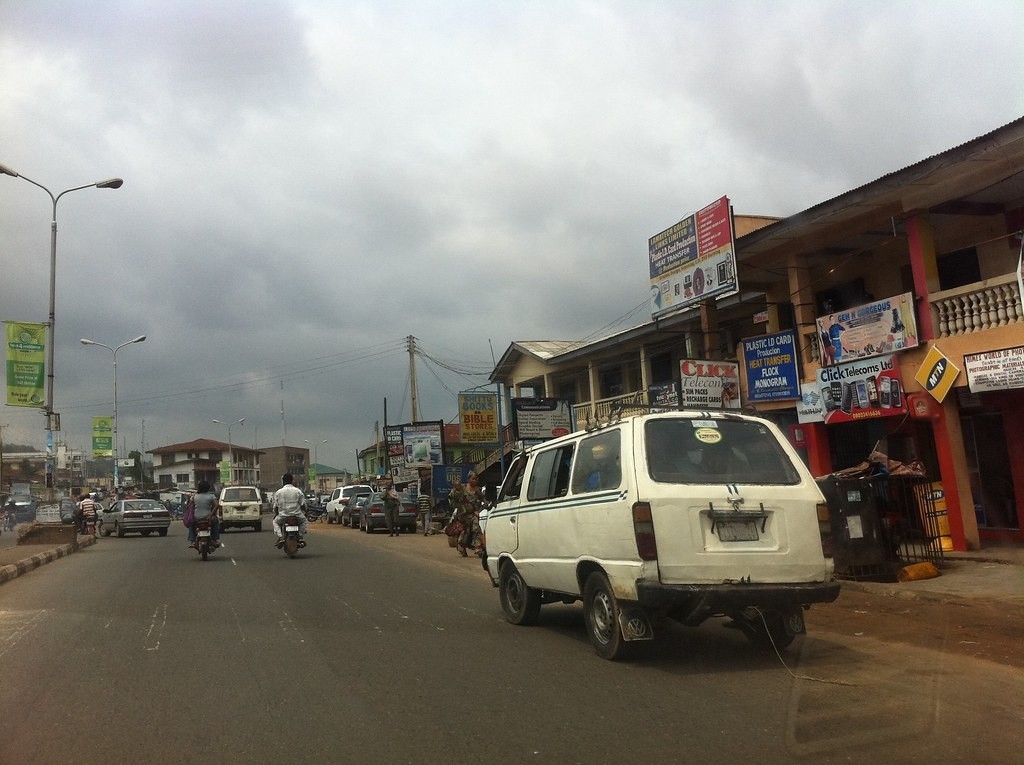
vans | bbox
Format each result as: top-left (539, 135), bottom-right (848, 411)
top-left (89, 491), bottom-right (104, 501)
top-left (479, 408), bottom-right (840, 661)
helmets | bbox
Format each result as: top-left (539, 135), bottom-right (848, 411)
top-left (84, 493), bottom-right (90, 498)
top-left (80, 493), bottom-right (84, 499)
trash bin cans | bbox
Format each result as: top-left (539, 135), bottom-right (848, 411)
top-left (817, 477), bottom-right (899, 582)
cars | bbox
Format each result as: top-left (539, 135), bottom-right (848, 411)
top-left (76, 503), bottom-right (104, 519)
top-left (309, 495), bottom-right (331, 505)
top-left (341, 494), bottom-right (370, 528)
top-left (481, 485), bottom-right (516, 503)
top-left (358, 493), bottom-right (418, 533)
top-left (98, 499), bottom-right (171, 537)
top-left (107, 489), bottom-right (117, 496)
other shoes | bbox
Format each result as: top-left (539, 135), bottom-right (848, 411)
top-left (274, 538), bottom-right (284, 547)
top-left (460, 550), bottom-right (468, 557)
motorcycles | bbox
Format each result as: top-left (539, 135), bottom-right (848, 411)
top-left (304, 503), bottom-right (328, 523)
top-left (72, 503), bottom-right (97, 539)
top-left (282, 515), bottom-right (306, 558)
top-left (192, 519), bottom-right (219, 560)
top-left (2, 511), bottom-right (16, 531)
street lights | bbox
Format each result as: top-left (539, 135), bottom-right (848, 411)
top-left (305, 439), bottom-right (328, 503)
top-left (1, 165), bottom-right (124, 521)
top-left (81, 335), bottom-right (146, 500)
top-left (212, 417), bottom-right (246, 486)
top-left (67, 448), bottom-right (79, 496)
top-left (350, 452), bottom-right (361, 485)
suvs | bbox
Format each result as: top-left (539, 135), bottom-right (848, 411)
top-left (218, 486), bottom-right (263, 532)
top-left (5, 495), bottom-right (42, 520)
top-left (323, 485), bottom-right (375, 523)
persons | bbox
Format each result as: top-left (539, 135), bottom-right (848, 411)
top-left (272, 473), bottom-right (308, 548)
top-left (675, 285), bottom-right (679, 296)
top-left (706, 274), bottom-right (712, 285)
top-left (817, 318), bottom-right (836, 366)
top-left (187, 480), bottom-right (221, 548)
top-left (93, 494), bottom-right (100, 503)
top-left (72, 493), bottom-right (98, 533)
top-left (418, 487), bottom-right (432, 536)
top-left (448, 470), bottom-right (497, 557)
top-left (898, 293), bottom-right (916, 341)
top-left (388, 443), bottom-right (402, 456)
top-left (4, 498), bottom-right (18, 525)
top-left (828, 315), bottom-right (847, 360)
top-left (379, 486), bottom-right (400, 536)
top-left (720, 371), bottom-right (739, 408)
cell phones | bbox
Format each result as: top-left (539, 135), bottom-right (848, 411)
top-left (821, 375), bottom-right (902, 415)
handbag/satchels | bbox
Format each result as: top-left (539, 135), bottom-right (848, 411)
top-left (183, 494), bottom-right (196, 528)
